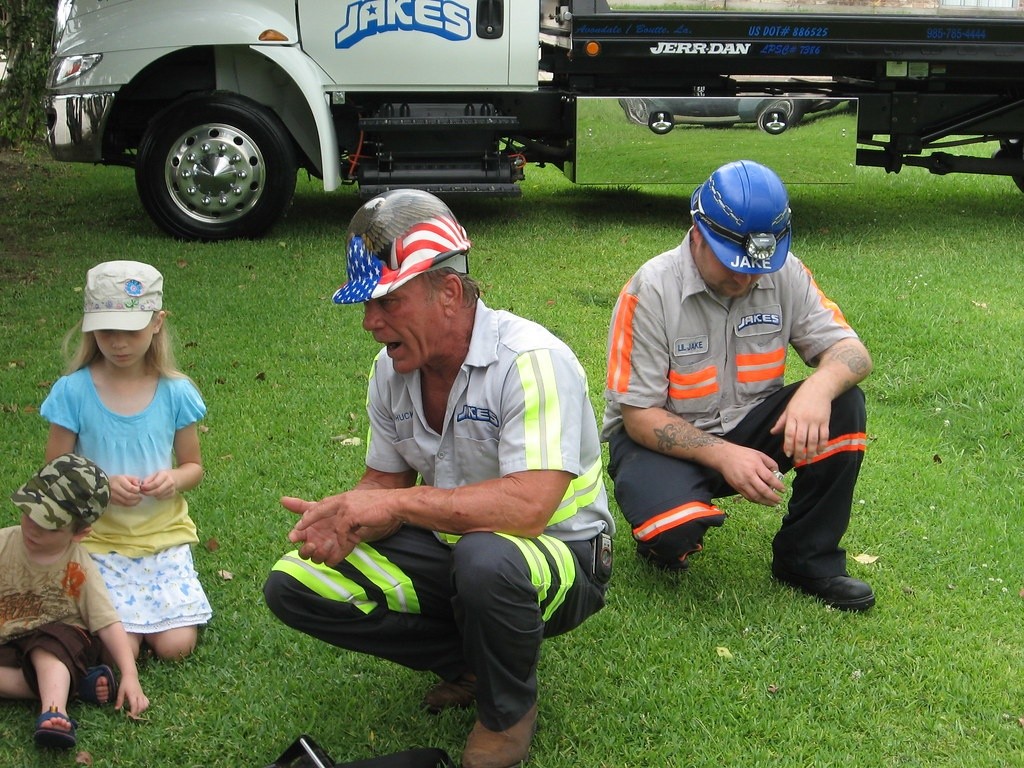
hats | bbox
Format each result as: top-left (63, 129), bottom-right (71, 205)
top-left (10, 453), bottom-right (111, 529)
top-left (80, 260), bottom-right (163, 332)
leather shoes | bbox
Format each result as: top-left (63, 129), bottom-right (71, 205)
top-left (463, 705), bottom-right (540, 768)
top-left (636, 544), bottom-right (690, 572)
top-left (771, 557), bottom-right (875, 610)
top-left (419, 668), bottom-right (478, 715)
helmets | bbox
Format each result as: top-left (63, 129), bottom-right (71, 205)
top-left (332, 187), bottom-right (472, 304)
top-left (691, 160), bottom-right (793, 273)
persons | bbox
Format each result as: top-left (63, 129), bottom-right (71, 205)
top-left (262, 188), bottom-right (616, 768)
top-left (599, 159), bottom-right (876, 612)
top-left (40, 259), bottom-right (214, 669)
top-left (1, 452), bottom-right (150, 750)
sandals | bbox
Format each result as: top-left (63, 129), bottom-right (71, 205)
top-left (34, 711), bottom-right (76, 749)
top-left (74, 665), bottom-right (116, 703)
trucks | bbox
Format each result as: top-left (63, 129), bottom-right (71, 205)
top-left (45, 0), bottom-right (1024, 242)
